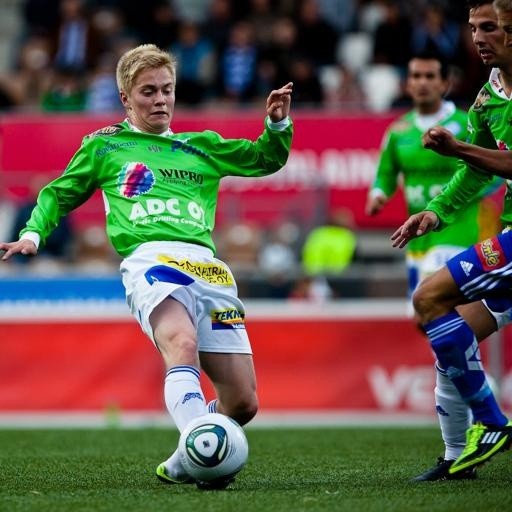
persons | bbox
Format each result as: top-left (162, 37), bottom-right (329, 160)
top-left (1, 2), bottom-right (511, 304)
top-left (408, 1), bottom-right (511, 474)
top-left (365, 45), bottom-right (503, 318)
top-left (2, 43), bottom-right (295, 490)
top-left (386, 2), bottom-right (511, 486)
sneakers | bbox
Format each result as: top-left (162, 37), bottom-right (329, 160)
top-left (448, 416), bottom-right (512, 476)
top-left (155, 447), bottom-right (192, 484)
top-left (410, 457), bottom-right (478, 481)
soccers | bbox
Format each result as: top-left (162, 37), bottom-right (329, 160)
top-left (177, 413), bottom-right (249, 482)
